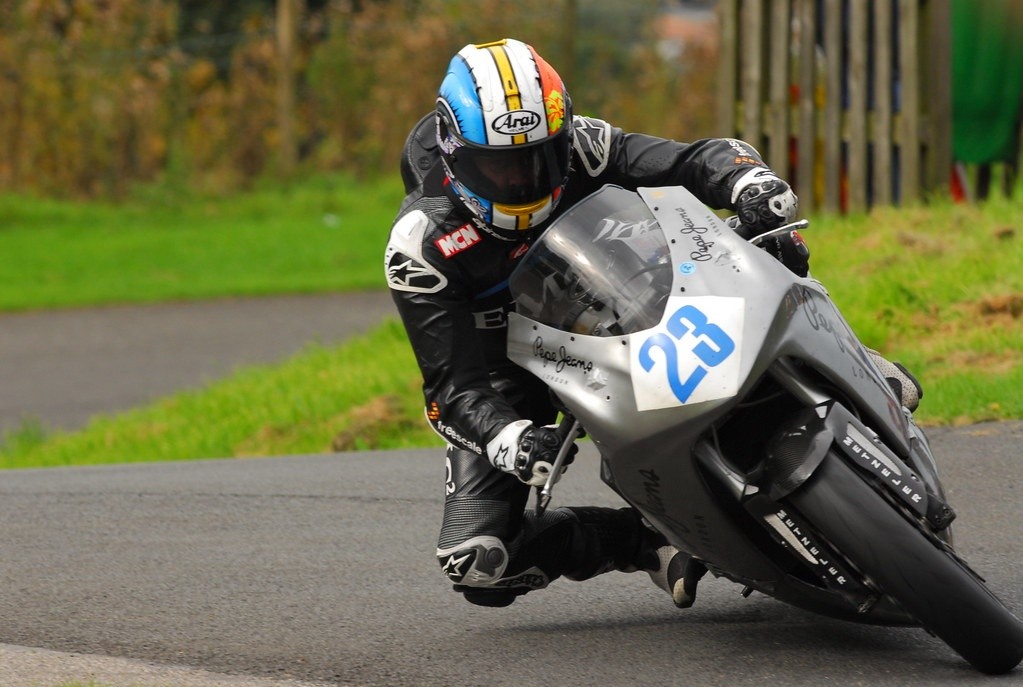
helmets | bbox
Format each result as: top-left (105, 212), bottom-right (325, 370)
top-left (435, 38), bottom-right (575, 241)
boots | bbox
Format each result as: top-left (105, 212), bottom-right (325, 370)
top-left (868, 346), bottom-right (919, 413)
top-left (554, 506), bottom-right (698, 608)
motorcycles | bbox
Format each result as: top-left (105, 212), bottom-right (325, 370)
top-left (490, 179), bottom-right (1022, 678)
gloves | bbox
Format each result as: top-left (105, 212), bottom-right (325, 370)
top-left (484, 419), bottom-right (587, 488)
top-left (727, 165), bottom-right (800, 246)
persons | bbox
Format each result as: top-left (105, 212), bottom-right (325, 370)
top-left (383, 39), bottom-right (798, 608)
